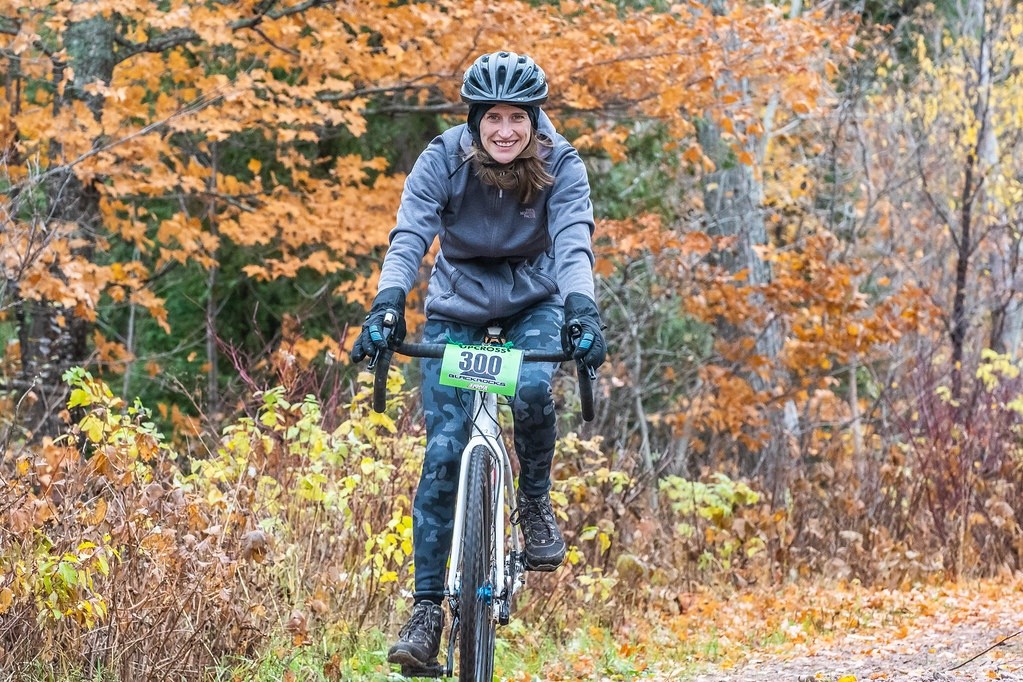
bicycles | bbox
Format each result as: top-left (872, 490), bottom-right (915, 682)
top-left (367, 308), bottom-right (608, 682)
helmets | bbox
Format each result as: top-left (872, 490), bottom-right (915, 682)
top-left (459, 51), bottom-right (549, 105)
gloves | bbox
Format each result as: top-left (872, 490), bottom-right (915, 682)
top-left (351, 287), bottom-right (407, 364)
top-left (564, 293), bottom-right (607, 371)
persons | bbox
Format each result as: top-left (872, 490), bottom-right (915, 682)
top-left (350, 50), bottom-right (610, 666)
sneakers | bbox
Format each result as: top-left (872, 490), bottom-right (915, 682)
top-left (388, 600), bottom-right (445, 667)
top-left (509, 486), bottom-right (566, 571)
